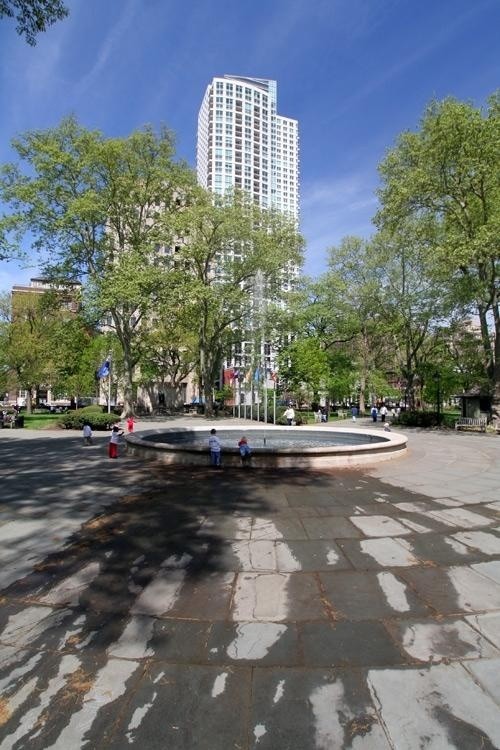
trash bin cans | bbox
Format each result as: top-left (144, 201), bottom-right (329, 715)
top-left (197, 404), bottom-right (204, 414)
top-left (14, 416), bottom-right (25, 428)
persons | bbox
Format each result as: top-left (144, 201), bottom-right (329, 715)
top-left (207, 428), bottom-right (226, 467)
top-left (31, 400), bottom-right (69, 414)
top-left (371, 410), bottom-right (378, 422)
top-left (379, 404), bottom-right (388, 422)
top-left (370, 406), bottom-right (378, 421)
top-left (0, 405), bottom-right (20, 429)
top-left (396, 407), bottom-right (401, 418)
top-left (213, 399), bottom-right (219, 417)
top-left (237, 435), bottom-right (253, 460)
top-left (351, 405), bottom-right (358, 423)
top-left (108, 426), bottom-right (125, 459)
top-left (383, 420), bottom-right (392, 433)
top-left (82, 420), bottom-right (94, 446)
top-left (283, 404), bottom-right (296, 426)
top-left (126, 415), bottom-right (136, 433)
top-left (391, 407), bottom-right (396, 418)
top-left (311, 400), bottom-right (329, 423)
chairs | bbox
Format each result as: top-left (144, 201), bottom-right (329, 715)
top-left (314, 411), bottom-right (327, 423)
top-left (337, 410), bottom-right (347, 421)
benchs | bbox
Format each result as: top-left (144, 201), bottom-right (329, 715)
top-left (455, 416), bottom-right (487, 433)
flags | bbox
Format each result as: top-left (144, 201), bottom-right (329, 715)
top-left (223, 365), bottom-right (280, 384)
top-left (96, 356), bottom-right (110, 380)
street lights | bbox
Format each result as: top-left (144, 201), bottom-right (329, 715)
top-left (432, 371), bottom-right (441, 423)
